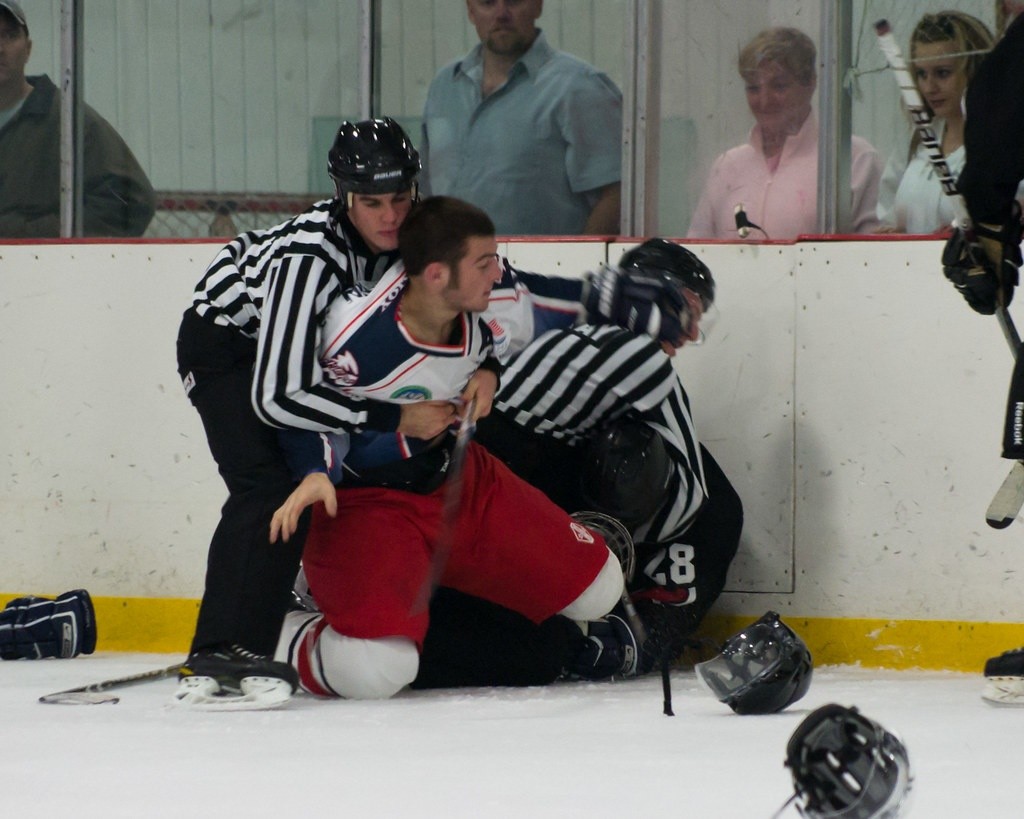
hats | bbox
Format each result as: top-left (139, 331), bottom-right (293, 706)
top-left (1, 0), bottom-right (26, 27)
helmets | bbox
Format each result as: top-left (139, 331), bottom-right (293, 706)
top-left (702, 610), bottom-right (813, 716)
top-left (619, 237), bottom-right (715, 313)
top-left (575, 416), bottom-right (670, 527)
top-left (326, 116), bottom-right (421, 196)
top-left (784, 705), bottom-right (909, 819)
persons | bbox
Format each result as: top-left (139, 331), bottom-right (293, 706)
top-left (420, 0), bottom-right (622, 235)
top-left (940, 1), bottom-right (1024, 316)
top-left (0, 0), bottom-right (158, 239)
top-left (882, 9), bottom-right (995, 234)
top-left (683, 26), bottom-right (884, 239)
top-left (268, 194), bottom-right (702, 699)
top-left (176, 115), bottom-right (502, 711)
top-left (413, 237), bottom-right (744, 689)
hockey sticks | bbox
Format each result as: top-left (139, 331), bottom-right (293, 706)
top-left (37, 662), bottom-right (185, 705)
top-left (873, 18), bottom-right (1023, 530)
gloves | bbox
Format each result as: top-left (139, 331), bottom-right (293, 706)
top-left (584, 264), bottom-right (691, 347)
top-left (0, 588), bottom-right (97, 660)
top-left (941, 200), bottom-right (1024, 316)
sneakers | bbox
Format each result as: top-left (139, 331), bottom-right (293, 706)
top-left (980, 648), bottom-right (1024, 707)
top-left (175, 638), bottom-right (300, 712)
